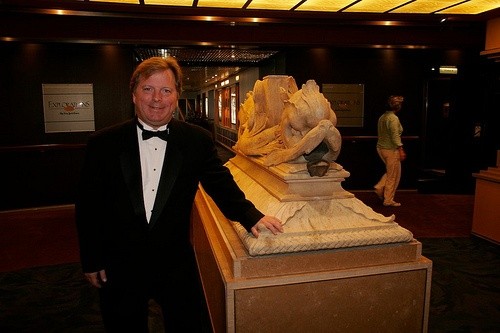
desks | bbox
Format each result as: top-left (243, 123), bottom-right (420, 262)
top-left (472, 173), bottom-right (500, 245)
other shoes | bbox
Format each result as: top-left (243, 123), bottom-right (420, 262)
top-left (384, 201), bottom-right (401, 206)
top-left (375, 185), bottom-right (382, 199)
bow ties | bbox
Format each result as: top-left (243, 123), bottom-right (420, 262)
top-left (143, 129), bottom-right (169, 141)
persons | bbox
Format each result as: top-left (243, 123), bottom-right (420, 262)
top-left (374, 94), bottom-right (405, 205)
top-left (73, 56), bottom-right (284, 332)
